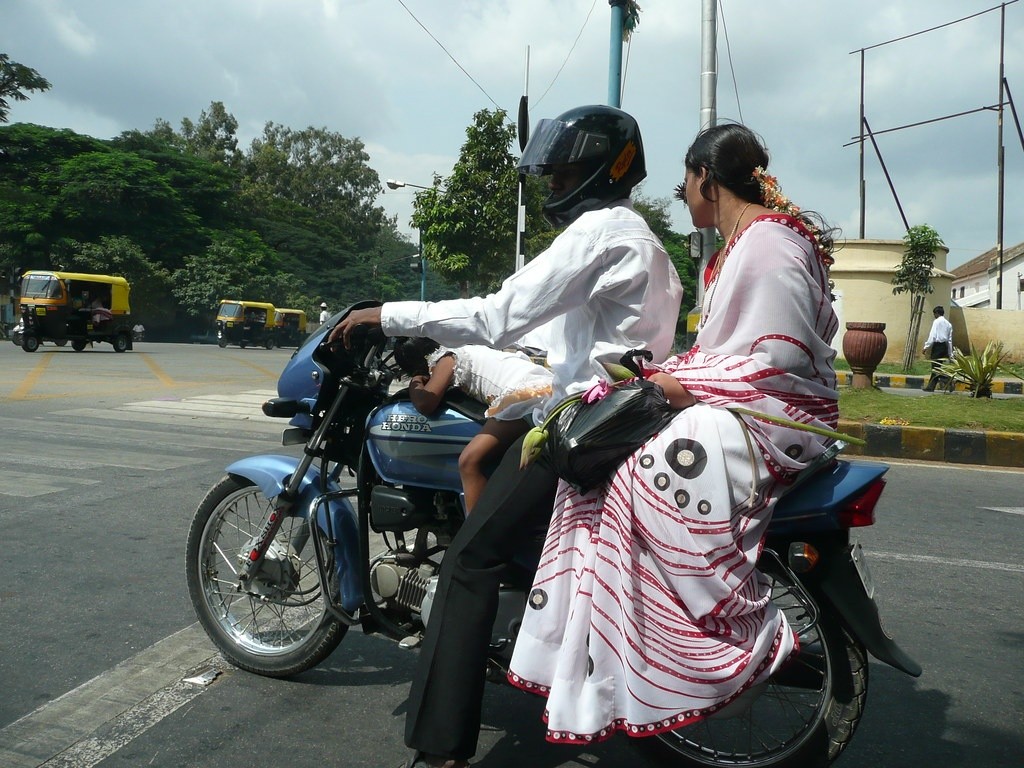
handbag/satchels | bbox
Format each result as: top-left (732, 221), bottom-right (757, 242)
top-left (536, 349), bottom-right (683, 497)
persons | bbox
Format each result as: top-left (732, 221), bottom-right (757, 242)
top-left (921, 306), bottom-right (953, 391)
top-left (325, 103), bottom-right (839, 768)
top-left (132, 320), bottom-right (145, 341)
top-left (319, 302), bottom-right (331, 326)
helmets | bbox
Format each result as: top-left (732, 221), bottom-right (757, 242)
top-left (536, 104), bottom-right (647, 229)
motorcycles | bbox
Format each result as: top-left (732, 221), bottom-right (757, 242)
top-left (274, 309), bottom-right (308, 348)
top-left (184, 300), bottom-right (924, 768)
top-left (14, 269), bottom-right (133, 353)
top-left (214, 297), bottom-right (276, 350)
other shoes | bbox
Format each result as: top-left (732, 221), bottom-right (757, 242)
top-left (922, 387), bottom-right (934, 392)
top-left (397, 749), bottom-right (468, 768)
